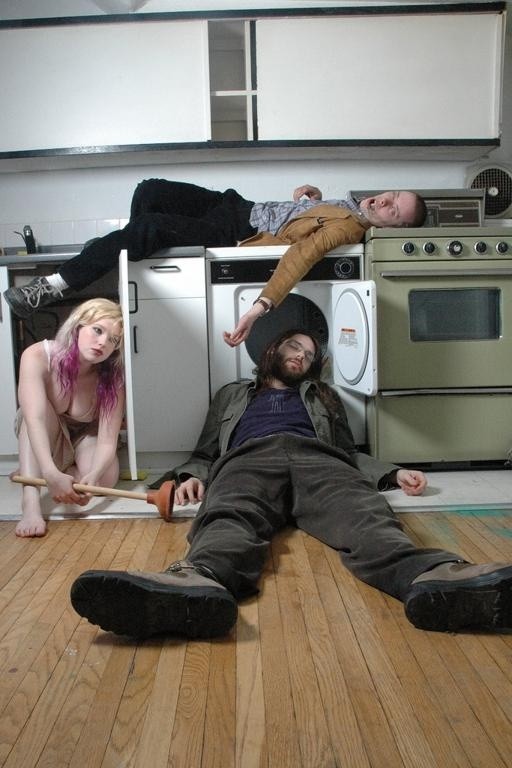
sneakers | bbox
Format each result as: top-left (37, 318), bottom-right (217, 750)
top-left (70, 558), bottom-right (238, 640)
top-left (3, 275), bottom-right (64, 319)
top-left (403, 557), bottom-right (512, 636)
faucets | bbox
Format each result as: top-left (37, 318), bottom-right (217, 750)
top-left (24, 225), bottom-right (36, 254)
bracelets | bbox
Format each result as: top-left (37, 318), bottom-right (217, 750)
top-left (253, 298), bottom-right (270, 317)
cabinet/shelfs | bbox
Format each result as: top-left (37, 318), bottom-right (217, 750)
top-left (129, 257), bottom-right (212, 470)
top-left (0, 247), bottom-right (139, 483)
top-left (0, 3), bottom-right (508, 176)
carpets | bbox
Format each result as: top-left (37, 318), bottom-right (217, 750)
top-left (0, 468), bottom-right (512, 522)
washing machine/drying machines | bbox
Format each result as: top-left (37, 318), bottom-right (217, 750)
top-left (206, 255), bottom-right (379, 446)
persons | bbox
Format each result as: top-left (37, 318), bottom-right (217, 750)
top-left (71, 328), bottom-right (512, 641)
top-left (13, 297), bottom-right (126, 538)
top-left (2, 179), bottom-right (428, 346)
